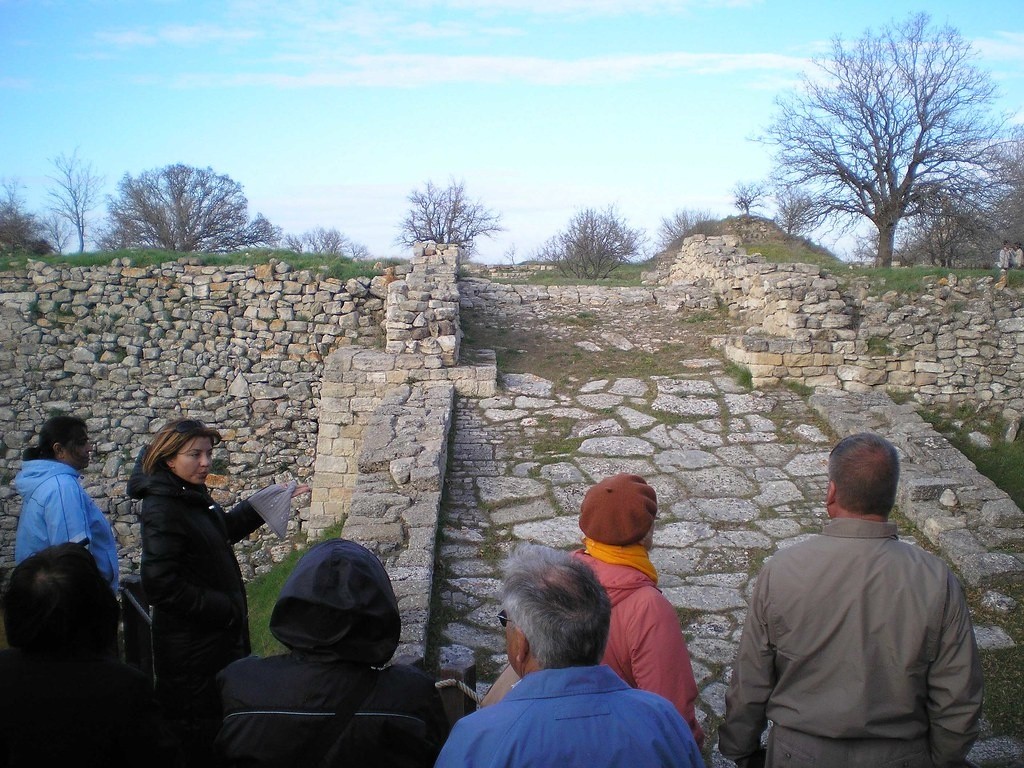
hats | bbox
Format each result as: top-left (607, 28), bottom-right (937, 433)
top-left (251, 482), bottom-right (296, 543)
top-left (579, 473), bottom-right (657, 547)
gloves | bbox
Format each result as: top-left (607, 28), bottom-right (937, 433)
top-left (736, 750), bottom-right (767, 768)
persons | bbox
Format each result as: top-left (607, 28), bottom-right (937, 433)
top-left (1, 540), bottom-right (215, 767)
top-left (996, 239), bottom-right (1024, 270)
top-left (215, 537), bottom-right (451, 767)
top-left (716, 436), bottom-right (983, 768)
top-left (568, 473), bottom-right (706, 748)
top-left (431, 543), bottom-right (706, 768)
top-left (128, 418), bottom-right (310, 740)
top-left (15, 415), bottom-right (119, 594)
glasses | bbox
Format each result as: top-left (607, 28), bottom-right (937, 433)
top-left (496, 608), bottom-right (512, 626)
